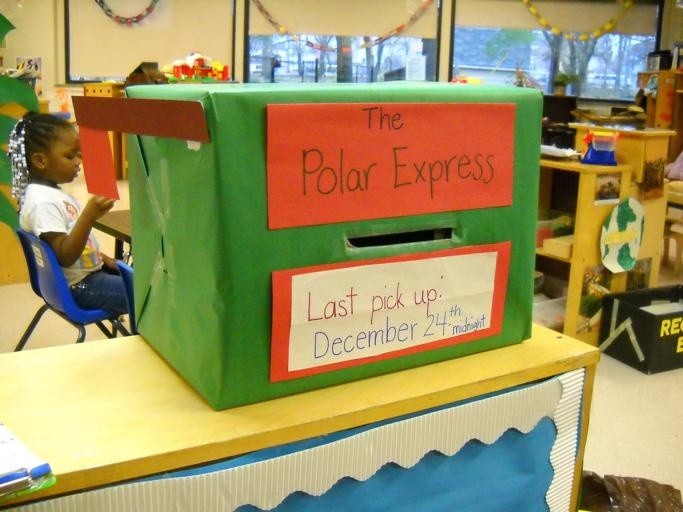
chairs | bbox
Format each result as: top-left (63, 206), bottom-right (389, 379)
top-left (15, 232), bottom-right (132, 352)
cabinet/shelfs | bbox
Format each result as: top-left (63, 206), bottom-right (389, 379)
top-left (533, 129), bottom-right (671, 355)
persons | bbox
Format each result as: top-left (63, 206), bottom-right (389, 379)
top-left (11, 112), bottom-right (134, 316)
